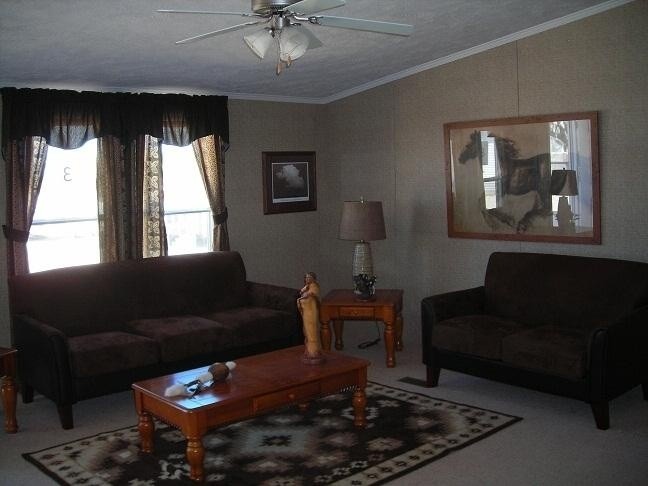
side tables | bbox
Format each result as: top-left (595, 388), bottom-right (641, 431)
top-left (319, 288), bottom-right (404, 368)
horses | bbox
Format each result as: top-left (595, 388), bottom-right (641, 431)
top-left (458, 130), bottom-right (567, 234)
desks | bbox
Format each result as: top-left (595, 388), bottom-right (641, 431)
top-left (1, 346), bottom-right (18, 435)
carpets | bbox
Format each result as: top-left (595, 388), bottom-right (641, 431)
top-left (21, 378), bottom-right (524, 486)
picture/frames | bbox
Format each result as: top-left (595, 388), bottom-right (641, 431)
top-left (261, 150), bottom-right (317, 216)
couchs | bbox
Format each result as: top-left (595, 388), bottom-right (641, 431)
top-left (419, 254), bottom-right (647, 430)
top-left (9, 253), bottom-right (302, 430)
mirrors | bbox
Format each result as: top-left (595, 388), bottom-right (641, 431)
top-left (443, 112), bottom-right (601, 244)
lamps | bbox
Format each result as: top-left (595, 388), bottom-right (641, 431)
top-left (548, 167), bottom-right (579, 232)
top-left (336, 195), bottom-right (387, 302)
top-left (244, 0), bottom-right (310, 74)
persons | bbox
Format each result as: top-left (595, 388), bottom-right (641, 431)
top-left (296, 271), bottom-right (326, 365)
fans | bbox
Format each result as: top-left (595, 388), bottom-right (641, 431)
top-left (156, 0), bottom-right (410, 51)
